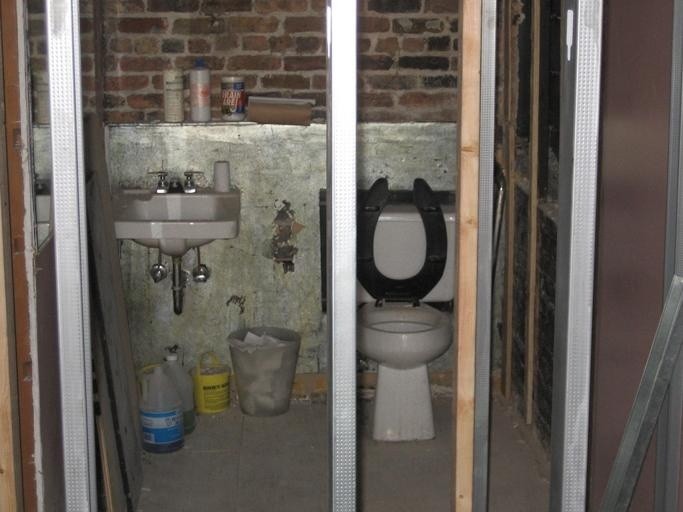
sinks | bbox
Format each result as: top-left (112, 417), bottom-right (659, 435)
top-left (33, 221), bottom-right (55, 251)
top-left (110, 185), bottom-right (242, 256)
top-left (35, 193), bottom-right (53, 224)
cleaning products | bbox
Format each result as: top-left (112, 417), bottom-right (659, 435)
top-left (161, 56), bottom-right (186, 123)
top-left (188, 58), bottom-right (213, 123)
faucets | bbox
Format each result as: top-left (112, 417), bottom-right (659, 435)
top-left (167, 178), bottom-right (184, 193)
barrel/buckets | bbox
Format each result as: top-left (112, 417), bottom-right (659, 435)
top-left (137, 364), bottom-right (187, 453)
top-left (163, 344), bottom-right (198, 435)
top-left (190, 349), bottom-right (231, 417)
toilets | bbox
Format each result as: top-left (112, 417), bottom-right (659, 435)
top-left (357, 177), bottom-right (457, 444)
